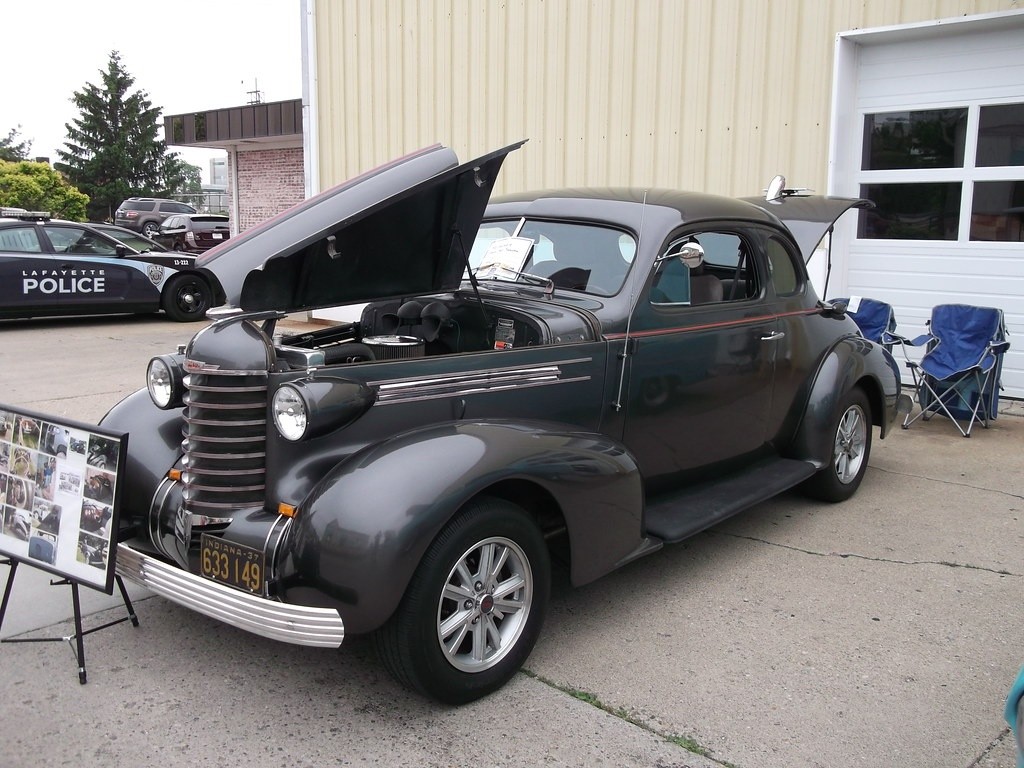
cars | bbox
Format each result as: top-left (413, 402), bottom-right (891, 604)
top-left (0, 206), bottom-right (225, 324)
top-left (84, 140), bottom-right (903, 708)
top-left (151, 213), bottom-right (230, 253)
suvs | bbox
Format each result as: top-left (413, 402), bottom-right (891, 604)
top-left (114, 197), bottom-right (198, 239)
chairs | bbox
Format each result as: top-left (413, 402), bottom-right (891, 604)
top-left (880, 304), bottom-right (1012, 438)
top-left (652, 236), bottom-right (724, 302)
top-left (941, 212), bottom-right (1024, 241)
top-left (826, 297), bottom-right (897, 354)
top-left (527, 230), bottom-right (599, 284)
